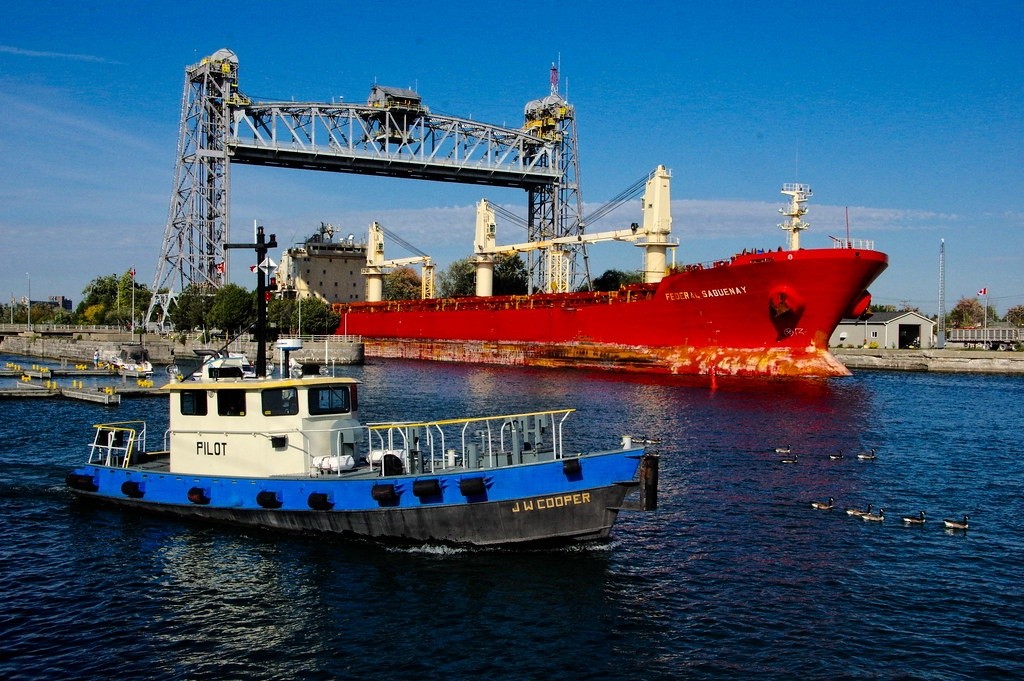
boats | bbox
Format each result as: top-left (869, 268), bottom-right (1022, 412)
top-left (61, 222), bottom-right (662, 550)
top-left (332, 164), bottom-right (892, 377)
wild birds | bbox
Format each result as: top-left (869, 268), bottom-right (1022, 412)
top-left (808, 495), bottom-right (971, 530)
top-left (770, 443), bottom-right (878, 466)
top-left (615, 434), bottom-right (664, 458)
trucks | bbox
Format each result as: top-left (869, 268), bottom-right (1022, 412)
top-left (950, 328), bottom-right (1024, 351)
